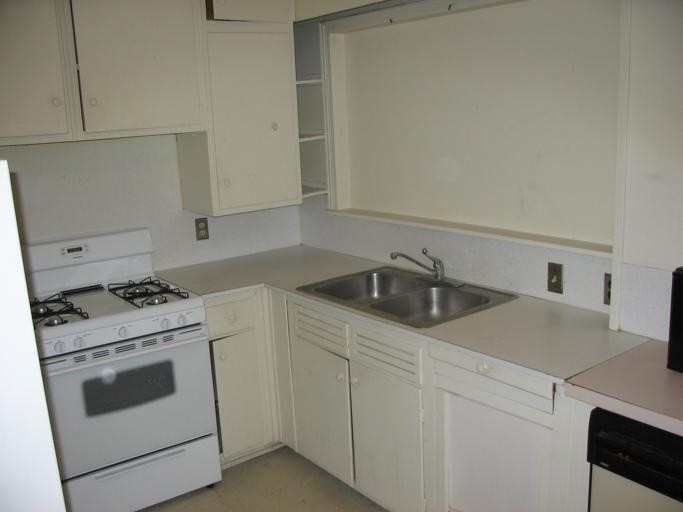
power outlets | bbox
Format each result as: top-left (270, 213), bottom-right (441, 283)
top-left (195, 216), bottom-right (208, 241)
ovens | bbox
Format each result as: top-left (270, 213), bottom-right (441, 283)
top-left (36, 323), bottom-right (225, 512)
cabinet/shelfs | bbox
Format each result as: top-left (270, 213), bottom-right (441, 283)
top-left (176, 29), bottom-right (302, 217)
top-left (205, 288), bottom-right (276, 465)
top-left (288, 295), bottom-right (425, 511)
top-left (0, 1), bottom-right (201, 142)
top-left (429, 345), bottom-right (568, 511)
top-left (295, 78), bottom-right (329, 198)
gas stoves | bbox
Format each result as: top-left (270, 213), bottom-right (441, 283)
top-left (23, 274), bottom-right (202, 331)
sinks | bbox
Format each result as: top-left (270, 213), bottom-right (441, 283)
top-left (366, 282), bottom-right (492, 327)
top-left (307, 265), bottom-right (423, 305)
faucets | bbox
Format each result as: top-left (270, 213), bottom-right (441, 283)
top-left (390, 244), bottom-right (447, 280)
